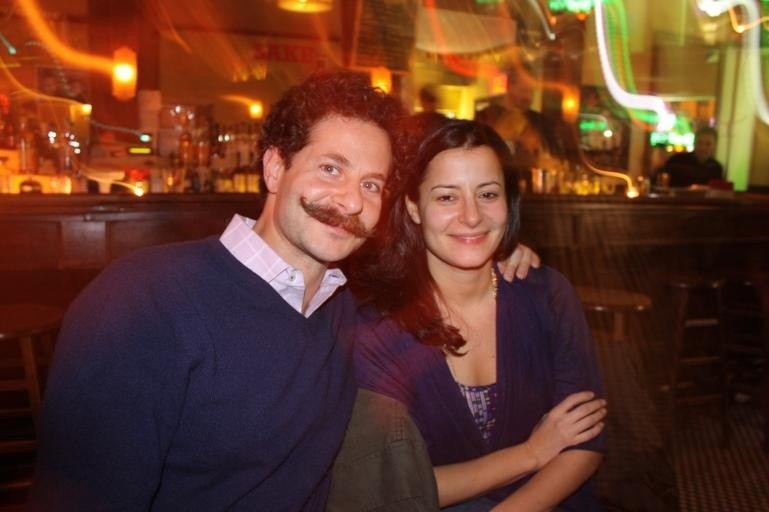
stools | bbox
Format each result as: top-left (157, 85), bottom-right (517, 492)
top-left (574, 263), bottom-right (769, 468)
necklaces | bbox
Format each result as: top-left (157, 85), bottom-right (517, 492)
top-left (489, 265), bottom-right (497, 300)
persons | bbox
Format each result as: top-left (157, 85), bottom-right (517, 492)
top-left (410, 79), bottom-right (450, 135)
top-left (651, 126), bottom-right (722, 188)
top-left (22, 70), bottom-right (541, 509)
top-left (475, 61), bottom-right (563, 152)
top-left (350, 120), bottom-right (609, 510)
